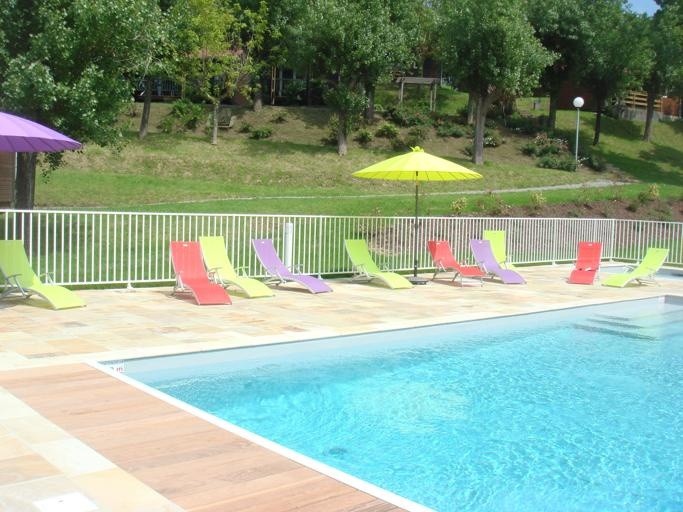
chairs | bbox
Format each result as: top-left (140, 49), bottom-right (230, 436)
top-left (470, 239), bottom-right (527, 284)
top-left (344, 239), bottom-right (415, 289)
top-left (0, 239), bottom-right (87, 310)
top-left (251, 239), bottom-right (334, 294)
top-left (567, 241), bottom-right (603, 284)
top-left (170, 241), bottom-right (233, 306)
top-left (429, 240), bottom-right (487, 288)
top-left (482, 230), bottom-right (515, 270)
top-left (602, 248), bottom-right (670, 287)
top-left (198, 235), bottom-right (275, 298)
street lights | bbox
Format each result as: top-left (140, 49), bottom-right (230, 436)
top-left (574, 97), bottom-right (585, 162)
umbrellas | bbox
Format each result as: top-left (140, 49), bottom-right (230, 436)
top-left (351, 145), bottom-right (484, 277)
top-left (0, 111), bottom-right (81, 157)
